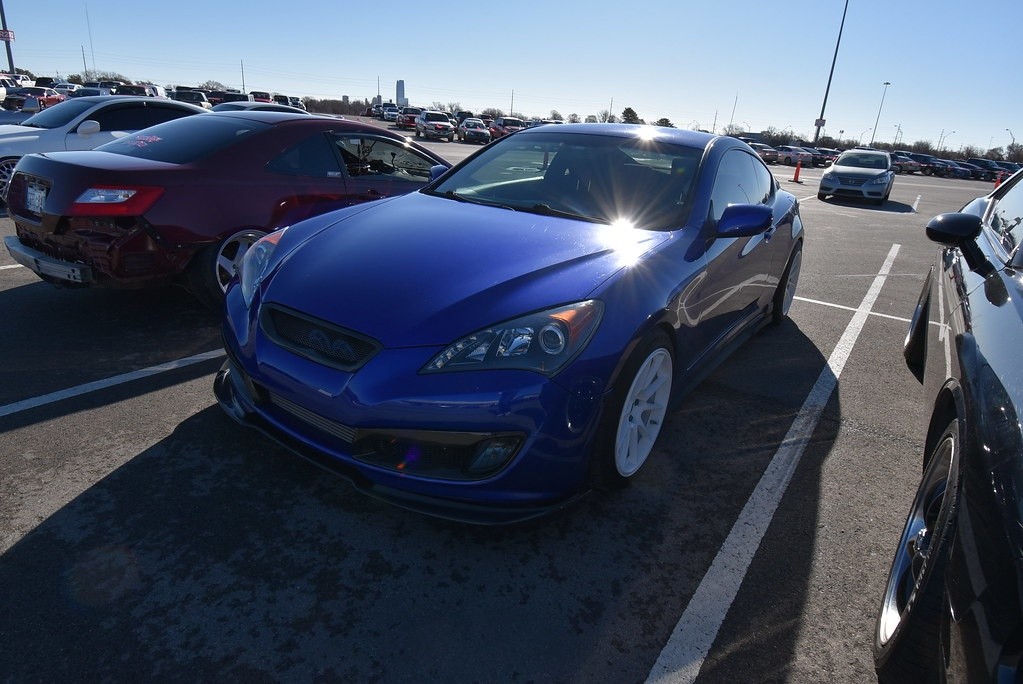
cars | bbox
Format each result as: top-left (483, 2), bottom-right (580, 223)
top-left (801, 146), bottom-right (842, 168)
top-left (872, 166), bottom-right (1023, 684)
top-left (2, 87), bottom-right (67, 110)
top-left (771, 145), bottom-right (814, 167)
top-left (890, 151), bottom-right (1023, 181)
top-left (746, 143), bottom-right (779, 165)
top-left (0, 72), bottom-right (308, 110)
top-left (0, 95), bottom-right (214, 207)
top-left (817, 148), bottom-right (900, 207)
top-left (3, 111), bottom-right (479, 320)
top-left (489, 117), bottom-right (527, 140)
top-left (371, 102), bottom-right (565, 131)
top-left (457, 119), bottom-right (490, 144)
top-left (208, 99), bottom-right (344, 119)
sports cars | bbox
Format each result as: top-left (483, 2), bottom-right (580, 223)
top-left (210, 121), bottom-right (806, 530)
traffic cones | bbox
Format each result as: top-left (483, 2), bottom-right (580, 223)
top-left (988, 175), bottom-right (1001, 196)
top-left (788, 159), bottom-right (805, 186)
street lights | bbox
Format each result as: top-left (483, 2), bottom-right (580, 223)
top-left (938, 130), bottom-right (956, 153)
top-left (1005, 129), bottom-right (1015, 160)
top-left (742, 121), bottom-right (750, 134)
top-left (780, 125), bottom-right (793, 135)
top-left (870, 82), bottom-right (891, 145)
top-left (893, 125), bottom-right (904, 146)
top-left (858, 127), bottom-right (872, 146)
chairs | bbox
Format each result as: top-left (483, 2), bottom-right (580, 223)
top-left (623, 169), bottom-right (680, 230)
top-left (874, 160), bottom-right (884, 167)
top-left (671, 158), bottom-right (697, 184)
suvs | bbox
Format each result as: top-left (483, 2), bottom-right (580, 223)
top-left (414, 111), bottom-right (455, 142)
